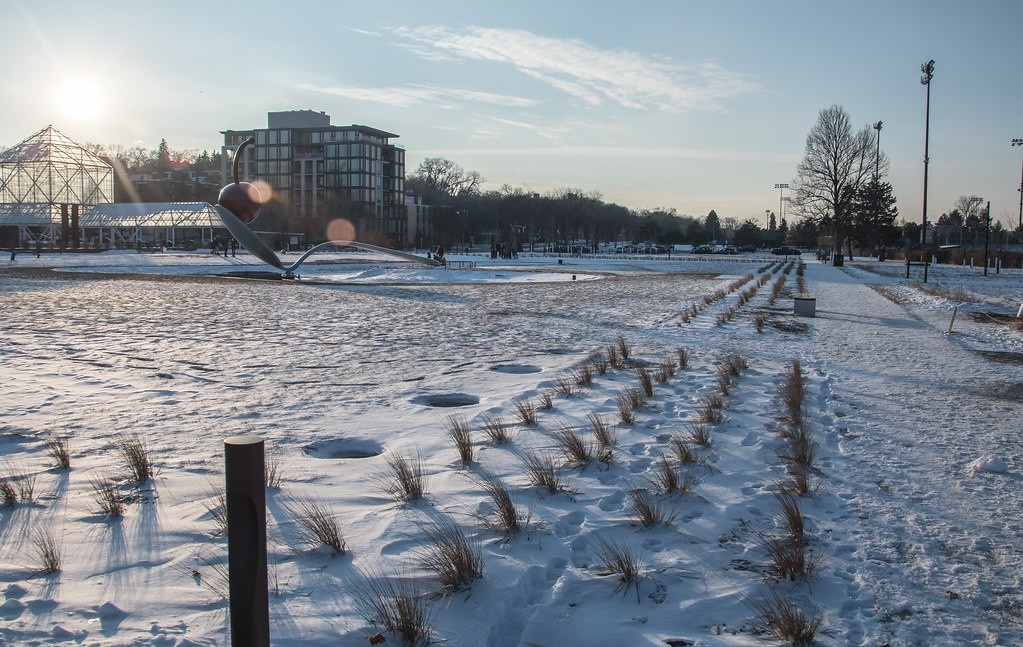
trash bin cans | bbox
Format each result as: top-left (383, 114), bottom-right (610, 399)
top-left (834, 255), bottom-right (844, 267)
top-left (491, 249), bottom-right (497, 259)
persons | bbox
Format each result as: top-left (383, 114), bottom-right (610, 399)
top-left (427, 241), bottom-right (446, 265)
top-left (383, 235), bottom-right (393, 249)
top-left (584, 240), bottom-right (599, 254)
top-left (211, 234), bottom-right (235, 257)
top-left (664, 241), bottom-right (671, 253)
top-left (491, 239), bottom-right (518, 260)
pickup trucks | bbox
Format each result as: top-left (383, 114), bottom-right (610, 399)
top-left (771, 247), bottom-right (803, 256)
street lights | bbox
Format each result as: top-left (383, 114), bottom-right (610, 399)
top-left (1011, 138), bottom-right (1023, 236)
top-left (873, 119), bottom-right (883, 236)
top-left (920, 57), bottom-right (938, 249)
top-left (775, 183), bottom-right (789, 233)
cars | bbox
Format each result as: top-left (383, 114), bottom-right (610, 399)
top-left (689, 243), bottom-right (757, 254)
top-left (551, 241), bottom-right (599, 254)
top-left (616, 243), bottom-right (674, 254)
